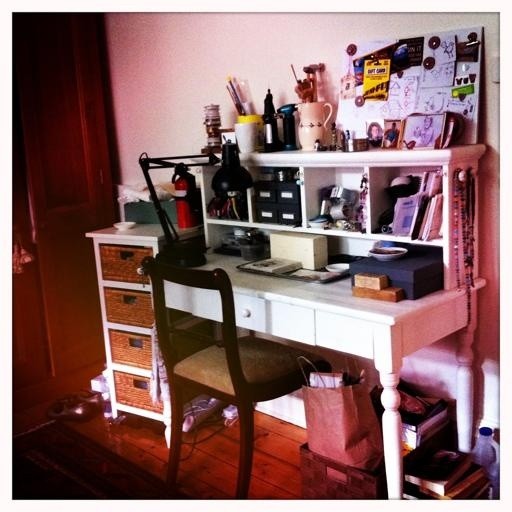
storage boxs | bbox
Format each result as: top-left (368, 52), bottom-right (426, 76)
top-left (124, 203), bottom-right (179, 224)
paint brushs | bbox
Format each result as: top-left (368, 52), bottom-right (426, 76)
top-left (225, 75), bottom-right (257, 116)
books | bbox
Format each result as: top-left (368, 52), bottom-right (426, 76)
top-left (403, 441), bottom-right (494, 498)
top-left (391, 168), bottom-right (441, 240)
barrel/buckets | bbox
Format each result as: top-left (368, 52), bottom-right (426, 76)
top-left (471, 426), bottom-right (500, 500)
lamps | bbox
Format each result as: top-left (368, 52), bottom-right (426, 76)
top-left (139, 143), bottom-right (254, 268)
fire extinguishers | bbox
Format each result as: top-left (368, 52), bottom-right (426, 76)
top-left (172, 162), bottom-right (199, 229)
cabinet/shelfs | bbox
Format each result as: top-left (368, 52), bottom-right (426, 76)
top-left (86, 222), bottom-right (180, 449)
top-left (192, 144), bottom-right (486, 500)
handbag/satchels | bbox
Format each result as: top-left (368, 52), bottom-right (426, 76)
top-left (296, 356), bottom-right (384, 484)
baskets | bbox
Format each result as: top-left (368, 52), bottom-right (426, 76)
top-left (103, 286), bottom-right (154, 328)
top-left (100, 245), bottom-right (152, 285)
top-left (112, 371), bottom-right (164, 415)
top-left (108, 329), bottom-right (153, 370)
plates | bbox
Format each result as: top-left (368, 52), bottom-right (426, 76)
top-left (325, 263), bottom-right (351, 273)
top-left (113, 221), bottom-right (136, 232)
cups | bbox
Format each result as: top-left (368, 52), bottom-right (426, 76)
top-left (351, 138), bottom-right (369, 151)
top-left (329, 203), bottom-right (352, 220)
top-left (237, 113), bottom-right (266, 153)
top-left (234, 121), bottom-right (258, 154)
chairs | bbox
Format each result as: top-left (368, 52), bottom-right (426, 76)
top-left (142, 258), bottom-right (332, 500)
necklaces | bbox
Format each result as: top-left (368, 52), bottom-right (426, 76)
top-left (452, 167), bottom-right (477, 326)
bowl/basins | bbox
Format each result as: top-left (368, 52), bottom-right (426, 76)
top-left (369, 247), bottom-right (409, 262)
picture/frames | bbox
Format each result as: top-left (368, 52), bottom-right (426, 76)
top-left (401, 112), bottom-right (446, 148)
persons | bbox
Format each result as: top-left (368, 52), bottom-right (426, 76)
top-left (416, 116), bottom-right (435, 147)
top-left (366, 120), bottom-right (383, 147)
top-left (384, 121), bottom-right (398, 148)
